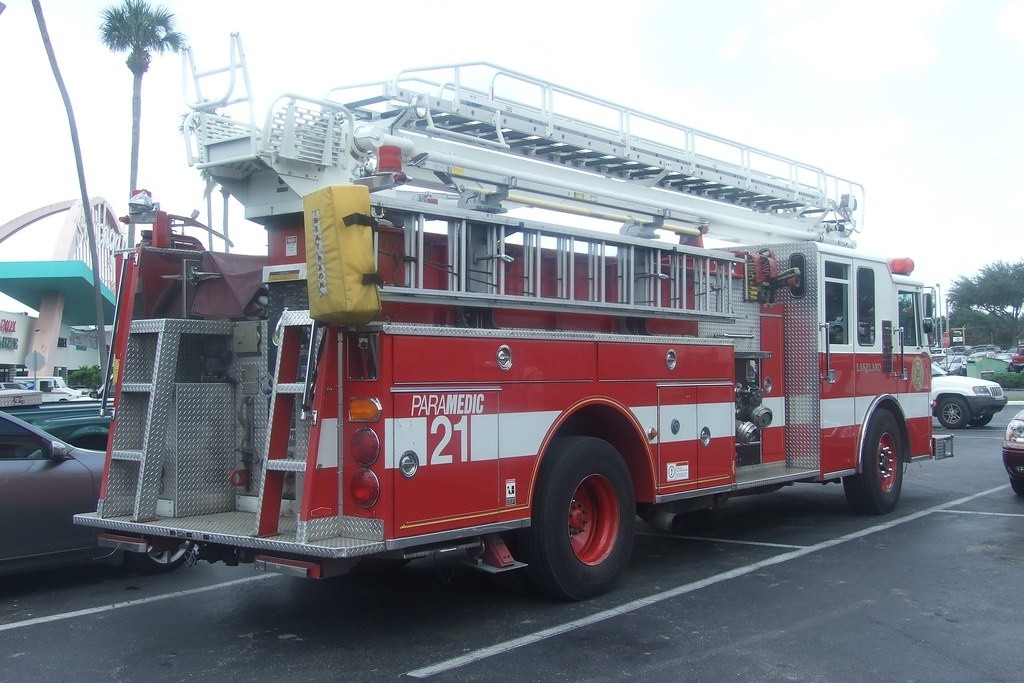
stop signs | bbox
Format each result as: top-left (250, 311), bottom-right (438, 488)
top-left (941, 332), bottom-right (950, 349)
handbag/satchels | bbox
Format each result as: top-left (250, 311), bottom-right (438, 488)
top-left (302, 184), bottom-right (382, 326)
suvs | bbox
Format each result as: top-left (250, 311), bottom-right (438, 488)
top-left (1012, 344), bottom-right (1024, 372)
top-left (930, 361), bottom-right (1008, 428)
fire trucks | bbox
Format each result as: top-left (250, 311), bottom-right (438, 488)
top-left (72, 30), bottom-right (955, 602)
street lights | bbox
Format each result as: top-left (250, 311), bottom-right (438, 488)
top-left (946, 298), bottom-right (955, 334)
top-left (936, 283), bottom-right (942, 348)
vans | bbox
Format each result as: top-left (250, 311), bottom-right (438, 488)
top-left (14, 377), bottom-right (83, 401)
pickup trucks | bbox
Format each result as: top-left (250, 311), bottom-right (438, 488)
top-left (0, 388), bottom-right (114, 451)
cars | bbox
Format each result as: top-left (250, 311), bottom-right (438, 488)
top-left (930, 344), bottom-right (1017, 376)
top-left (1002, 408), bottom-right (1024, 497)
top-left (0, 410), bottom-right (194, 573)
